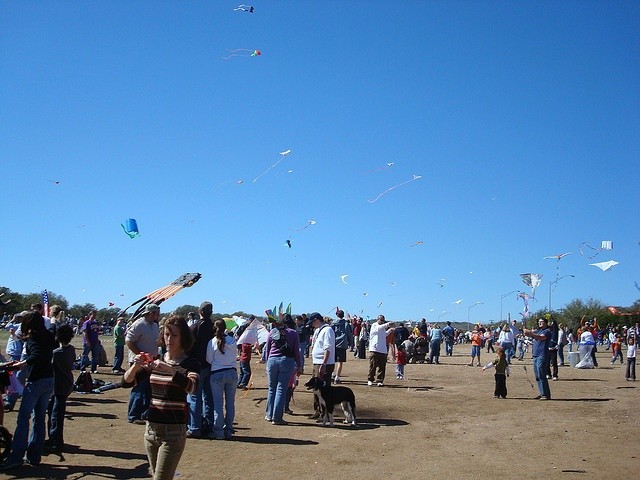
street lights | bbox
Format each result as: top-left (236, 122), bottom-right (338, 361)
top-left (270, 327), bottom-right (295, 357)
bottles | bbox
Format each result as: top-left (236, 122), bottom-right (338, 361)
top-left (306, 312), bottom-right (322, 325)
top-left (200, 301), bottom-right (213, 310)
top-left (141, 304), bottom-right (159, 316)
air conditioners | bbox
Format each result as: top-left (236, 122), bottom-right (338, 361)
top-left (304, 377), bottom-right (357, 428)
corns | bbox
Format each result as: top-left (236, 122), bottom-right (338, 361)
top-left (120, 218), bottom-right (141, 239)
top-left (279, 150), bottom-right (291, 157)
top-left (589, 260), bottom-right (619, 272)
top-left (237, 318), bottom-right (270, 353)
top-left (308, 219), bottom-right (316, 225)
top-left (174, 272), bottom-right (202, 287)
top-left (516, 291), bottom-right (535, 305)
top-left (282, 240), bottom-right (291, 248)
top-left (341, 275), bottom-right (348, 284)
top-left (601, 241), bottom-right (612, 250)
top-left (543, 252), bottom-right (572, 259)
top-left (520, 273), bottom-right (543, 292)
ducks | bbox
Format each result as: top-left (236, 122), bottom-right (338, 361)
top-left (92, 340), bottom-right (108, 367)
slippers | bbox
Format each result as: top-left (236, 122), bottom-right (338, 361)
top-left (75, 372), bottom-right (93, 392)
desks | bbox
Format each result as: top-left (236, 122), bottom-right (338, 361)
top-left (500, 291), bottom-right (520, 321)
top-left (549, 274), bottom-right (575, 311)
top-left (468, 302), bottom-right (484, 331)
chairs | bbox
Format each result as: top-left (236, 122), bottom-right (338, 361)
top-left (334, 379), bottom-right (341, 384)
top-left (208, 433), bottom-right (225, 439)
top-left (540, 395), bottom-right (551, 400)
top-left (368, 381), bottom-right (373, 386)
top-left (468, 363), bottom-right (473, 366)
top-left (23, 458), bottom-right (40, 466)
top-left (0, 455), bottom-right (23, 466)
top-left (477, 363), bottom-right (481, 366)
top-left (225, 434), bottom-right (231, 440)
top-left (283, 410), bottom-right (293, 415)
top-left (186, 431), bottom-right (204, 438)
top-left (128, 419), bottom-right (146, 425)
top-left (272, 420), bottom-right (287, 425)
top-left (265, 416), bottom-right (272, 421)
top-left (378, 382), bottom-right (384, 387)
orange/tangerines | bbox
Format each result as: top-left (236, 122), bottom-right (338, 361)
top-left (412, 336), bottom-right (431, 364)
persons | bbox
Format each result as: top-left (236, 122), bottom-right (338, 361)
top-left (491, 322), bottom-right (502, 336)
top-left (497, 323), bottom-right (515, 364)
top-left (401, 335), bottom-right (416, 364)
top-left (509, 320), bottom-right (522, 358)
top-left (121, 315), bottom-right (201, 480)
top-left (49, 304), bottom-right (64, 331)
top-left (429, 324), bottom-right (443, 364)
top-left (596, 322), bottom-right (640, 350)
top-left (577, 325), bottom-right (583, 350)
top-left (345, 313), bottom-right (362, 356)
top-left (610, 333), bottom-right (623, 364)
top-left (453, 328), bottom-right (473, 345)
top-left (416, 318), bottom-right (427, 340)
top-left (302, 313), bottom-right (312, 359)
top-left (0, 312), bottom-right (15, 327)
top-left (357, 322), bottom-right (368, 359)
top-left (523, 317), bottom-right (552, 400)
top-left (3, 311), bottom-right (31, 411)
top-left (108, 317), bottom-right (115, 335)
top-left (522, 336), bottom-right (531, 353)
top-left (57, 311), bottom-right (88, 336)
top-left (1, 310), bottom-right (56, 470)
top-left (294, 316), bottom-right (306, 375)
top-left (557, 323), bottom-right (567, 366)
top-left (265, 313), bottom-right (303, 426)
top-left (625, 328), bottom-right (637, 381)
top-left (394, 323), bottom-right (410, 345)
top-left (544, 319), bottom-right (558, 381)
top-left (515, 334), bottom-right (529, 361)
top-left (43, 325), bottom-right (76, 452)
top-left (486, 328), bottom-right (495, 353)
top-left (124, 304), bottom-right (164, 424)
top-left (580, 327), bottom-right (598, 367)
top-left (79, 310), bottom-right (100, 374)
top-left (468, 324), bottom-right (484, 367)
top-left (204, 319), bottom-right (239, 440)
top-left (185, 302), bottom-right (216, 439)
top-left (112, 317), bottom-right (128, 373)
top-left (30, 303), bottom-right (51, 330)
top-left (412, 322), bottom-right (421, 338)
top-left (568, 329), bottom-right (575, 351)
top-left (305, 312), bottom-right (335, 423)
top-left (395, 345), bottom-right (407, 379)
top-left (367, 315), bottom-right (398, 387)
top-left (442, 322), bottom-right (454, 356)
top-left (482, 346), bottom-right (510, 399)
top-left (4, 314), bottom-right (24, 376)
top-left (237, 341), bottom-right (259, 389)
top-left (100, 317), bottom-right (108, 336)
top-left (330, 310), bottom-right (354, 385)
top-left (187, 312), bottom-right (198, 327)
top-left (580, 315), bottom-right (599, 366)
